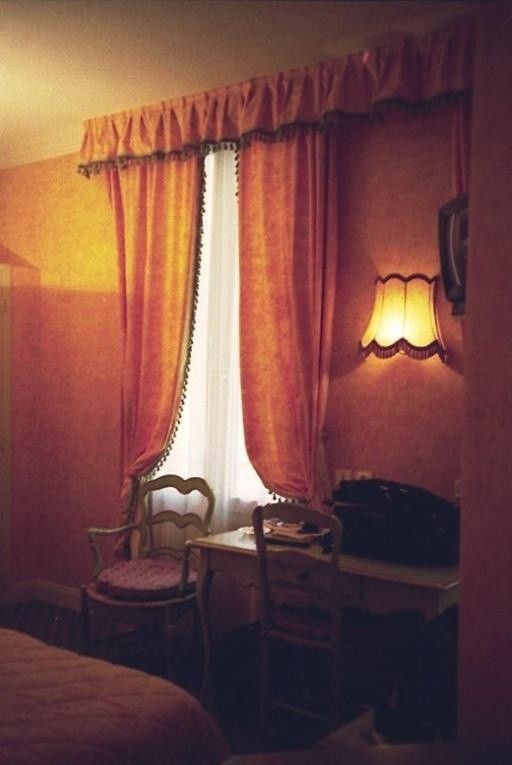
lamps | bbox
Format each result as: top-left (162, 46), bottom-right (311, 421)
top-left (356, 273), bottom-right (450, 364)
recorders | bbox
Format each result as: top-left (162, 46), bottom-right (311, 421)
top-left (326, 479), bottom-right (459, 569)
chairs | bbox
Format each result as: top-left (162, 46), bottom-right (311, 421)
top-left (77, 474), bottom-right (218, 686)
top-left (251, 502), bottom-right (397, 741)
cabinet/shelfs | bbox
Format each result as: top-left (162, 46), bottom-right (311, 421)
top-left (185, 524), bottom-right (462, 727)
